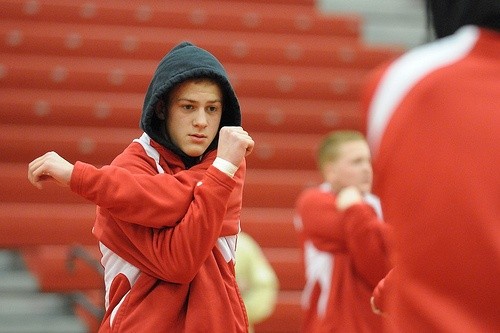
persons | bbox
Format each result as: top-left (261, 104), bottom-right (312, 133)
top-left (227, 229), bottom-right (281, 333)
top-left (26, 40), bottom-right (256, 333)
top-left (295, 126), bottom-right (394, 333)
top-left (363, 0), bottom-right (500, 333)
